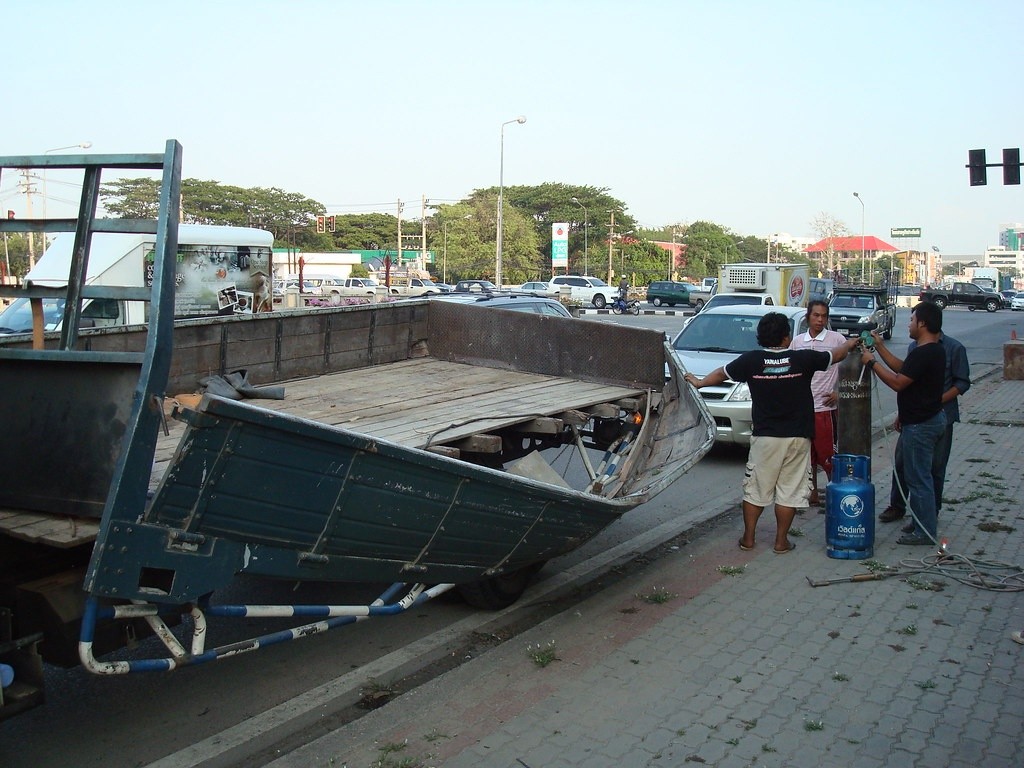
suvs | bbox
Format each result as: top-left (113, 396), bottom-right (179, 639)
top-left (646, 280), bottom-right (700, 309)
top-left (409, 276), bottom-right (621, 319)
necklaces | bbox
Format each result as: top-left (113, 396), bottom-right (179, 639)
top-left (767, 347), bottom-right (786, 349)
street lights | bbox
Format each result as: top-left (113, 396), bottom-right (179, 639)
top-left (42, 142), bottom-right (91, 254)
top-left (853, 192), bottom-right (865, 283)
top-left (571, 198), bottom-right (588, 276)
top-left (767, 233), bottom-right (778, 264)
top-left (495, 117), bottom-right (526, 290)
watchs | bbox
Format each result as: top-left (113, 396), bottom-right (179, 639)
top-left (866, 359), bottom-right (878, 370)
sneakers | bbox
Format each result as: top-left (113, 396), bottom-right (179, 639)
top-left (896, 534), bottom-right (937, 545)
top-left (901, 524), bottom-right (915, 533)
top-left (879, 506), bottom-right (906, 521)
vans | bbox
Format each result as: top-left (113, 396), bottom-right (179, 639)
top-left (809, 278), bottom-right (833, 304)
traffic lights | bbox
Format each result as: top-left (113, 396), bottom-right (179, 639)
top-left (316, 216), bottom-right (325, 233)
top-left (328, 215), bottom-right (335, 233)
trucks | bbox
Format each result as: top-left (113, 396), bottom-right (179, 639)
top-left (0, 224), bottom-right (274, 336)
top-left (825, 269), bottom-right (899, 343)
top-left (972, 268), bottom-right (1001, 293)
top-left (684, 263), bottom-right (809, 331)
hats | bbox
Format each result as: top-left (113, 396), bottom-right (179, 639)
top-left (622, 275), bottom-right (626, 278)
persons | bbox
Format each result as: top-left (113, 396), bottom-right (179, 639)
top-left (617, 275), bottom-right (629, 300)
top-left (861, 301), bottom-right (971, 544)
top-left (788, 300), bottom-right (847, 512)
top-left (685, 311), bottom-right (860, 555)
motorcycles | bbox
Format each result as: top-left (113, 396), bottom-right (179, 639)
top-left (612, 294), bottom-right (641, 316)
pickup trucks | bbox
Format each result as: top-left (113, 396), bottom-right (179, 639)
top-left (688, 282), bottom-right (717, 311)
top-left (918, 282), bottom-right (1002, 312)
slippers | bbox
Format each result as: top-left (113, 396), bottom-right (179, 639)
top-left (773, 542), bottom-right (796, 553)
top-left (808, 499), bottom-right (820, 506)
top-left (739, 537), bottom-right (752, 550)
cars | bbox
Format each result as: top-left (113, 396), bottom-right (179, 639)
top-left (274, 276), bottom-right (496, 294)
top-left (1001, 291), bottom-right (1024, 311)
top-left (664, 303), bottom-right (833, 444)
